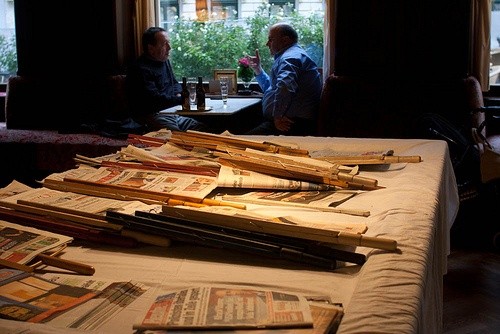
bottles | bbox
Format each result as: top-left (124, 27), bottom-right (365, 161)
top-left (196, 76), bottom-right (206, 109)
top-left (181, 77), bottom-right (190, 110)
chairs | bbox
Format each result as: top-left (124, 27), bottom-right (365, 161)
top-left (315, 75), bottom-right (347, 136)
top-left (5, 76), bottom-right (32, 130)
top-left (107, 73), bottom-right (131, 125)
top-left (466, 76), bottom-right (500, 184)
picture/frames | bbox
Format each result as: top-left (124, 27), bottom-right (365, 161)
top-left (214, 69), bottom-right (238, 95)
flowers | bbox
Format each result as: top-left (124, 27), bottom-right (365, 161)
top-left (239, 57), bottom-right (250, 68)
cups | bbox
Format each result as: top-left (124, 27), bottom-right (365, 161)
top-left (190, 93), bottom-right (196, 102)
top-left (220, 82), bottom-right (228, 104)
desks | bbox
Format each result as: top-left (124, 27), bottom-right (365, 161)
top-left (0, 135), bottom-right (459, 334)
top-left (158, 97), bottom-right (262, 117)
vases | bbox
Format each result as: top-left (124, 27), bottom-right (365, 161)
top-left (237, 67), bottom-right (256, 90)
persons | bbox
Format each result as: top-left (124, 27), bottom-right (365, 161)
top-left (125, 27), bottom-right (202, 134)
top-left (245, 23), bottom-right (322, 138)
